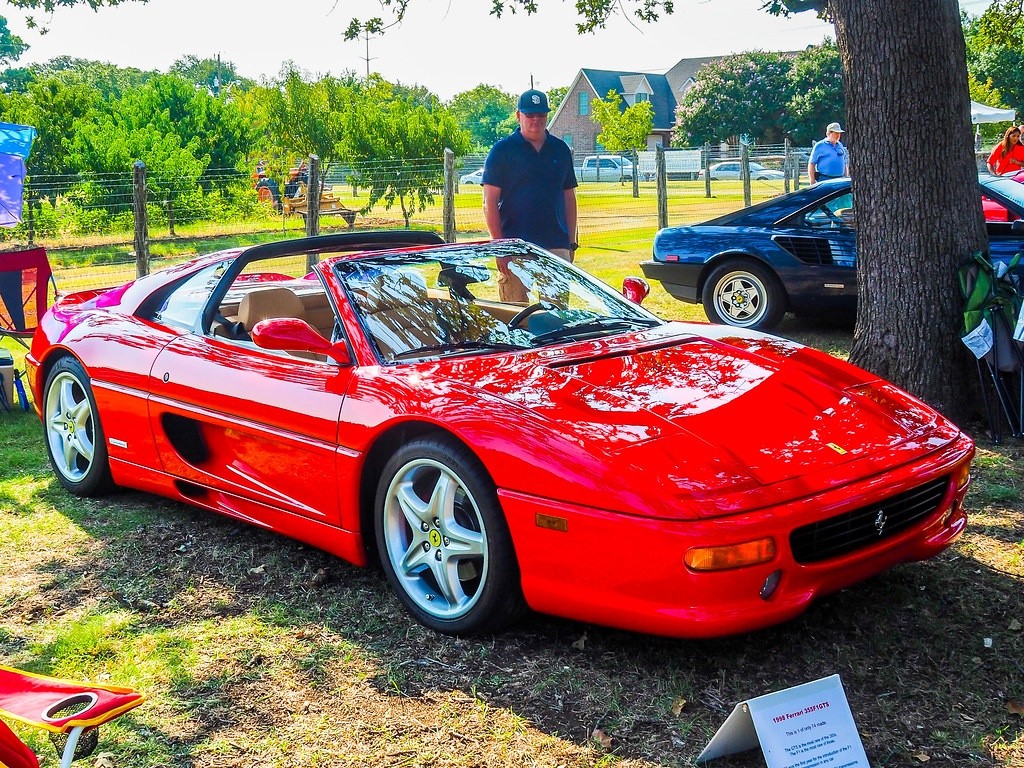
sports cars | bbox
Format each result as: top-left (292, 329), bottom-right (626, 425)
top-left (637, 172), bottom-right (1024, 333)
top-left (25, 220), bottom-right (975, 641)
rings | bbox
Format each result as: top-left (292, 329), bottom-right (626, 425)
top-left (1011, 159), bottom-right (1015, 163)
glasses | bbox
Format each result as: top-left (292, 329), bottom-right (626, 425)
top-left (525, 113), bottom-right (548, 117)
top-left (835, 148), bottom-right (845, 156)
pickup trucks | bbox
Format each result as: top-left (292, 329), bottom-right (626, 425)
top-left (571, 155), bottom-right (638, 183)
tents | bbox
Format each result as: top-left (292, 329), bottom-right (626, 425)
top-left (970, 99), bottom-right (1015, 124)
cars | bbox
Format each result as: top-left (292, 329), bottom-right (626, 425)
top-left (460, 169), bottom-right (484, 186)
top-left (698, 161), bottom-right (784, 181)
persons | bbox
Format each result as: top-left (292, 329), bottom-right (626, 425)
top-left (986, 126), bottom-right (1024, 176)
top-left (808, 122), bottom-right (849, 185)
top-left (480, 88), bottom-right (579, 310)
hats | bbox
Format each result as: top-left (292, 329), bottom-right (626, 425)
top-left (518, 89), bottom-right (552, 113)
top-left (827, 122), bottom-right (845, 133)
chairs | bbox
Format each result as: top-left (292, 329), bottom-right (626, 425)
top-left (238, 289), bottom-right (328, 363)
top-left (364, 272), bottom-right (443, 360)
top-left (831, 208), bottom-right (855, 229)
top-left (0, 247), bottom-right (56, 383)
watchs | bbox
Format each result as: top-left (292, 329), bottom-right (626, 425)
top-left (570, 243), bottom-right (578, 251)
